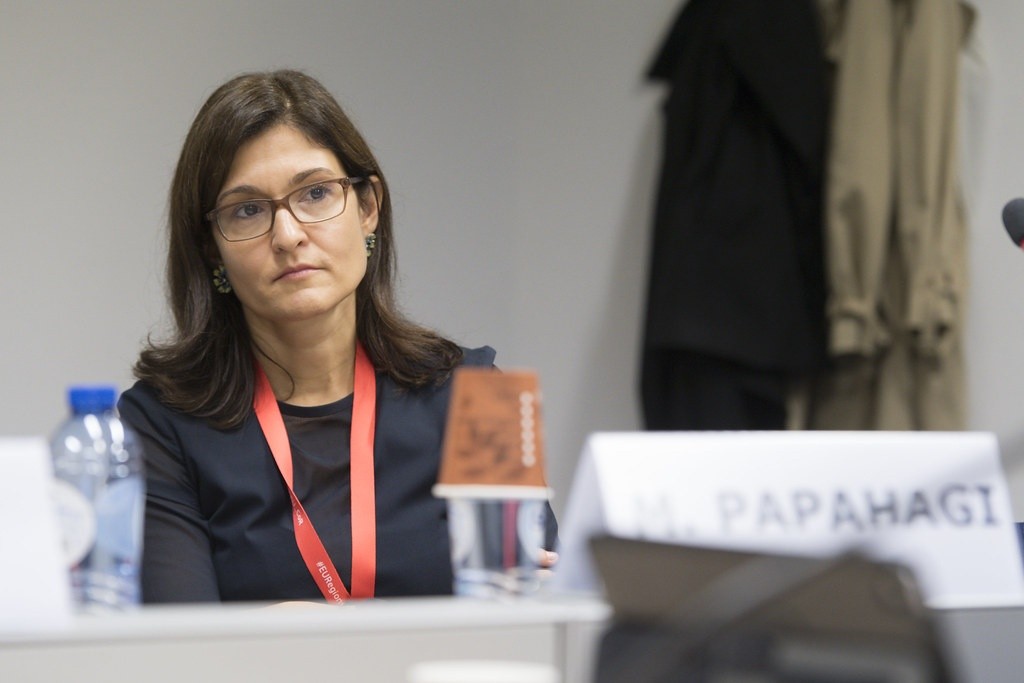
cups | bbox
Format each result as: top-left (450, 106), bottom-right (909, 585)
top-left (428, 365), bottom-right (554, 504)
top-left (442, 496), bottom-right (546, 597)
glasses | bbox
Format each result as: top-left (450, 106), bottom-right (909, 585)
top-left (206, 177), bottom-right (362, 242)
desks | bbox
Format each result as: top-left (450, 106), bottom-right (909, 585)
top-left (0, 591), bottom-right (1024, 683)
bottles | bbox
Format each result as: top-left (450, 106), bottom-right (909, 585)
top-left (46, 385), bottom-right (145, 605)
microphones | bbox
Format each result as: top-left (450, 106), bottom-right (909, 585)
top-left (1003, 198), bottom-right (1024, 252)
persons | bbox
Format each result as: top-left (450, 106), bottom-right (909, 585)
top-left (114, 67), bottom-right (560, 591)
